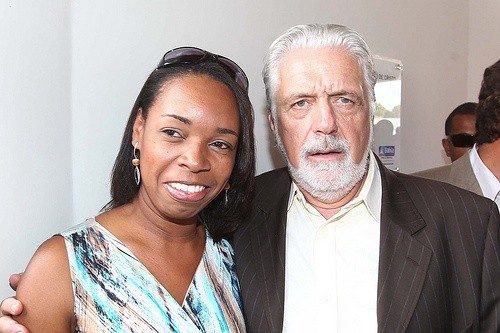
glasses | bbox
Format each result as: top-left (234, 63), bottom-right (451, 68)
top-left (447, 132), bottom-right (476, 148)
top-left (154, 46), bottom-right (249, 93)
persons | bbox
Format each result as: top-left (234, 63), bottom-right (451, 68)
top-left (406, 60), bottom-right (500, 208)
top-left (0, 23), bottom-right (500, 333)
top-left (13, 46), bottom-right (255, 333)
